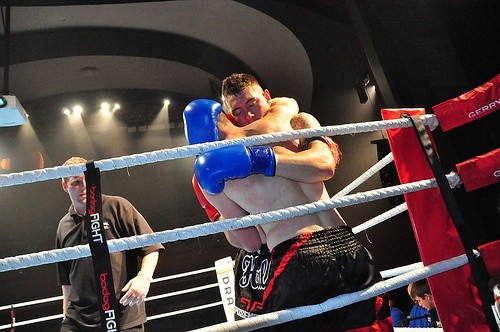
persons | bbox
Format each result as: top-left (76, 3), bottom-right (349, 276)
top-left (53, 157), bottom-right (165, 332)
top-left (181, 72), bottom-right (500, 332)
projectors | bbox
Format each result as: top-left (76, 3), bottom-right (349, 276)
top-left (0, 95), bottom-right (27, 127)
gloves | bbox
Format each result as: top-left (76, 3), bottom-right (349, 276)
top-left (194, 143), bottom-right (276, 195)
top-left (183, 99), bottom-right (222, 158)
